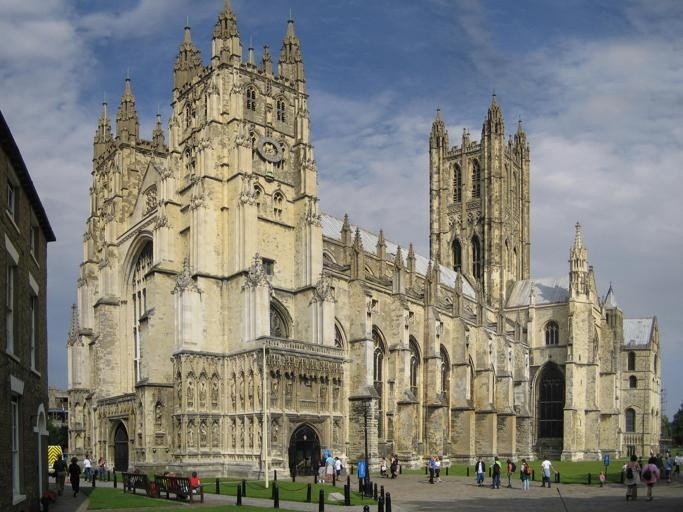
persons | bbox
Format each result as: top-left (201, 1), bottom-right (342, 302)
top-left (600, 452), bottom-right (680, 501)
top-left (98, 457), bottom-right (107, 482)
top-left (318, 455), bottom-right (326, 484)
top-left (541, 456), bottom-right (558, 488)
top-left (380, 453), bottom-right (398, 480)
top-left (127, 470), bottom-right (200, 500)
top-left (429, 455), bottom-right (442, 484)
top-left (84, 455), bottom-right (92, 484)
top-left (491, 457), bottom-right (501, 489)
top-left (507, 459), bottom-right (513, 488)
top-left (69, 458), bottom-right (81, 497)
top-left (521, 459), bottom-right (529, 491)
top-left (53, 455), bottom-right (69, 496)
top-left (326, 454), bottom-right (336, 483)
top-left (334, 457), bottom-right (342, 480)
top-left (475, 456), bottom-right (485, 487)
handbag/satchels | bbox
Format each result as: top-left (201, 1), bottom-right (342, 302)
top-left (494, 462), bottom-right (499, 474)
top-left (526, 467), bottom-right (531, 476)
top-left (643, 468), bottom-right (651, 480)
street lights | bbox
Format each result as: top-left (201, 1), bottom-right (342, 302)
top-left (360, 396), bottom-right (372, 497)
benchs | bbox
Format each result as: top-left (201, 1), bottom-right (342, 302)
top-left (152, 474), bottom-right (204, 504)
top-left (120, 472), bottom-right (153, 497)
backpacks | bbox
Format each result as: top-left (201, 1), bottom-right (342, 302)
top-left (510, 463), bottom-right (516, 472)
top-left (626, 466), bottom-right (633, 479)
